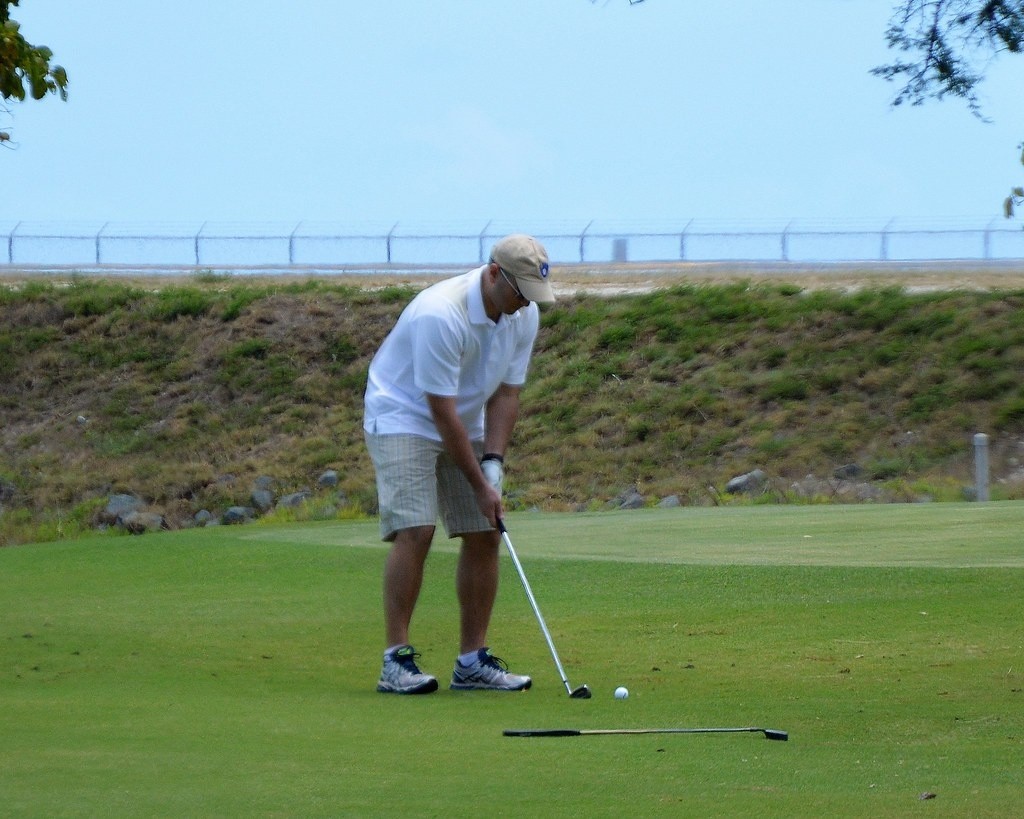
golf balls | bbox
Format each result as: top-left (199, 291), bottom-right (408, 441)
top-left (614, 686), bottom-right (629, 700)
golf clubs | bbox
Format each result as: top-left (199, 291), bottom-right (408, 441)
top-left (501, 727), bottom-right (789, 741)
top-left (495, 513), bottom-right (592, 699)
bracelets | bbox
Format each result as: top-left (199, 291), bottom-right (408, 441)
top-left (481, 452), bottom-right (503, 463)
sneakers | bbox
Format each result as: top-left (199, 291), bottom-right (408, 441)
top-left (449, 647), bottom-right (532, 691)
top-left (376, 646), bottom-right (439, 694)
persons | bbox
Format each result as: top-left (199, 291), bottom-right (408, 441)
top-left (363, 233), bottom-right (558, 695)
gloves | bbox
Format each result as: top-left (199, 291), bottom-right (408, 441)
top-left (480, 452), bottom-right (504, 499)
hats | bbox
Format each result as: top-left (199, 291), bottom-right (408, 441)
top-left (489, 234), bottom-right (556, 305)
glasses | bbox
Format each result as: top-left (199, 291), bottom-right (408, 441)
top-left (498, 268), bottom-right (526, 301)
